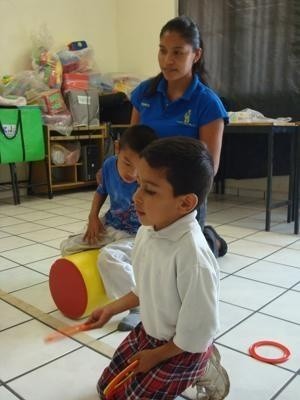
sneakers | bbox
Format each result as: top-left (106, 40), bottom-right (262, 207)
top-left (203, 224), bottom-right (229, 259)
top-left (192, 343), bottom-right (231, 400)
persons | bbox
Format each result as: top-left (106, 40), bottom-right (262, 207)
top-left (128, 14), bottom-right (228, 263)
top-left (60, 126), bottom-right (165, 331)
top-left (79, 135), bottom-right (233, 400)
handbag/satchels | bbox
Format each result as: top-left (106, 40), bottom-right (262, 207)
top-left (0, 107), bottom-right (47, 164)
top-left (64, 87), bottom-right (101, 128)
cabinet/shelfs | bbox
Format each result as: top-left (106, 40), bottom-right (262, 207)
top-left (28, 124), bottom-right (106, 198)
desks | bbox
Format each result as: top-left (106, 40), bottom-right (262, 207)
top-left (0, 105), bottom-right (46, 207)
top-left (214, 121), bottom-right (300, 232)
top-left (100, 122), bottom-right (133, 128)
